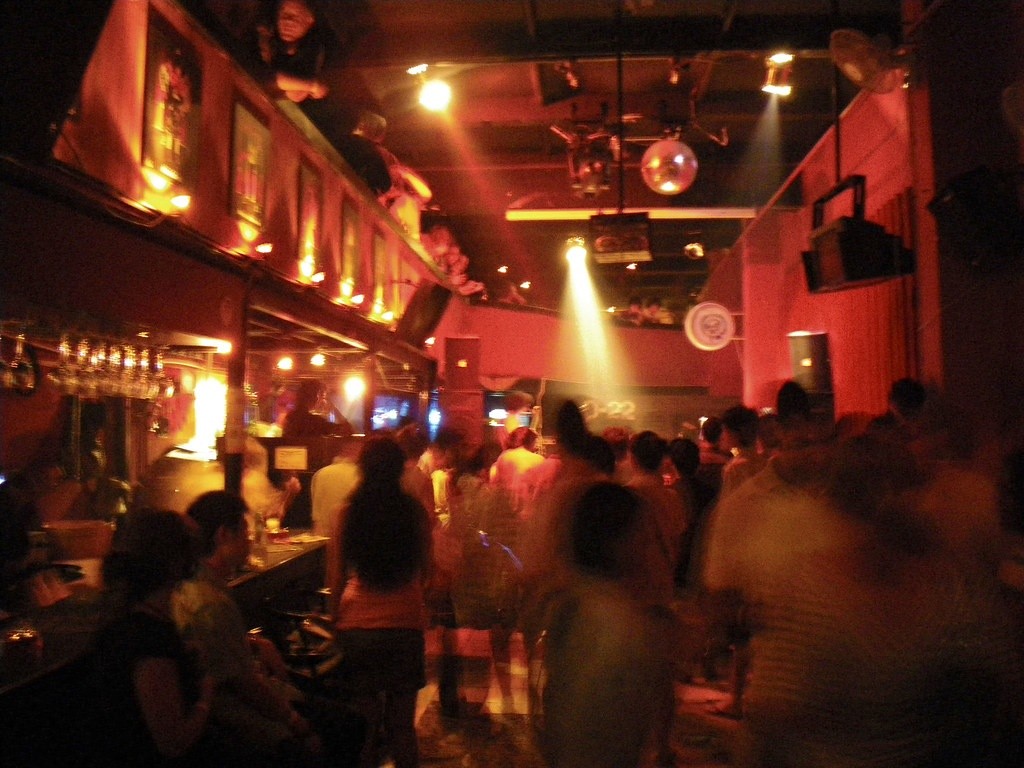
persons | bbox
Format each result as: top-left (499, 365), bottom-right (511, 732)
top-left (1, 366), bottom-right (1024, 768)
top-left (261, 0), bottom-right (329, 101)
top-left (621, 294), bottom-right (675, 325)
top-left (343, 109), bottom-right (487, 295)
